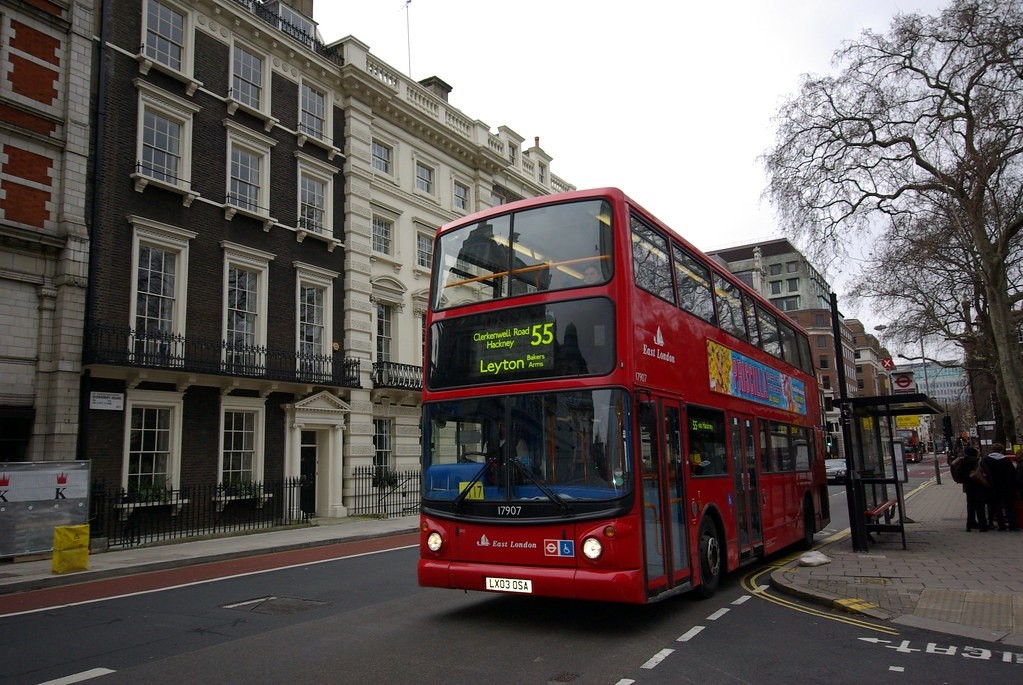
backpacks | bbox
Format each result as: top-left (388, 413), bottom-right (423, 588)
top-left (951, 458), bottom-right (968, 484)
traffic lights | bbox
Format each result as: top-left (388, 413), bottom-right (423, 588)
top-left (942, 415), bottom-right (952, 438)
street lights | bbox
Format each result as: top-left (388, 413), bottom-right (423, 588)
top-left (875, 324), bottom-right (942, 486)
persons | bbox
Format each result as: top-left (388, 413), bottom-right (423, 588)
top-left (480, 416), bottom-right (530, 486)
top-left (579, 265), bottom-right (603, 285)
top-left (953, 443), bottom-right (1023, 532)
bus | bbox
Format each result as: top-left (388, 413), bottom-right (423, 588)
top-left (895, 427), bottom-right (923, 463)
top-left (417, 186), bottom-right (831, 607)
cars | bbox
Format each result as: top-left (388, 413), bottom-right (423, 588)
top-left (824, 459), bottom-right (846, 484)
top-left (928, 440), bottom-right (949, 455)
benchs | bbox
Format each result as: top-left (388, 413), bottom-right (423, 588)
top-left (867, 498), bottom-right (897, 544)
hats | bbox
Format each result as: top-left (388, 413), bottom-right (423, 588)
top-left (965, 447), bottom-right (978, 458)
